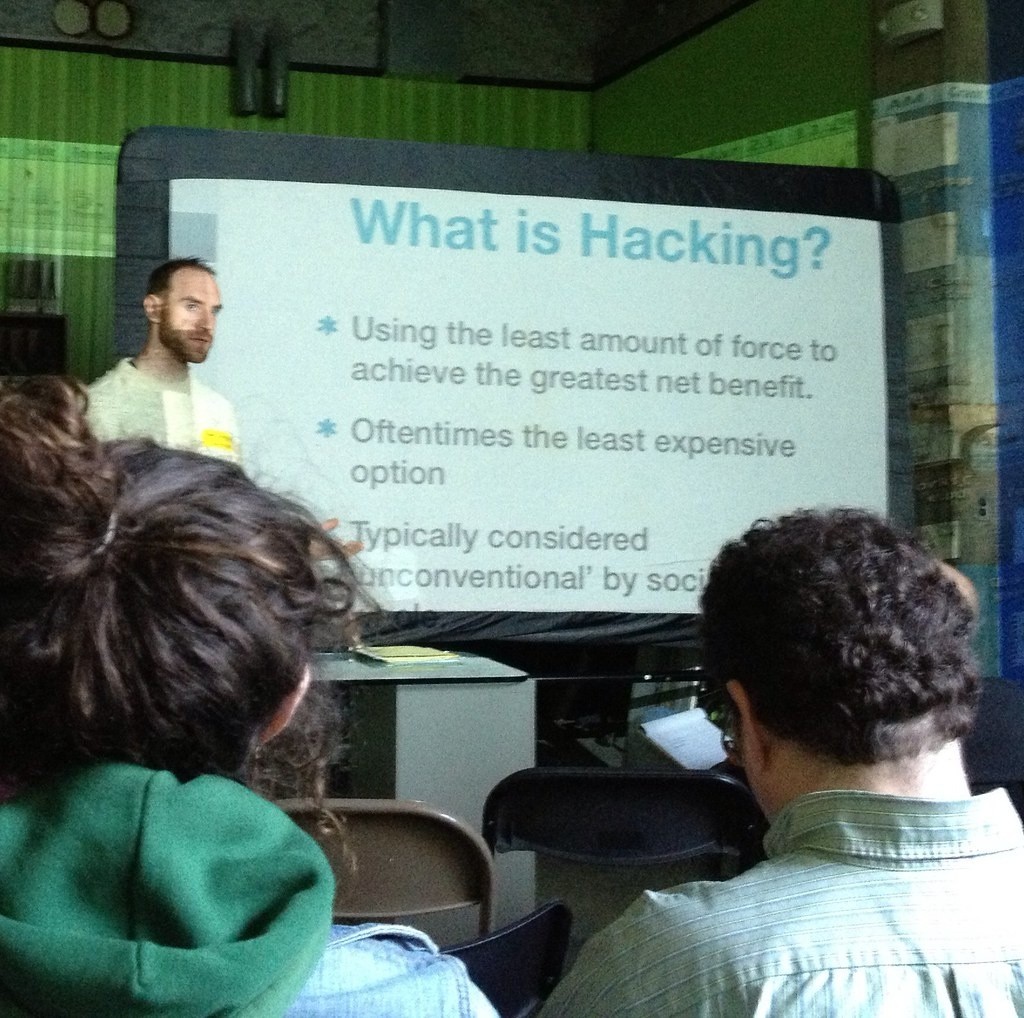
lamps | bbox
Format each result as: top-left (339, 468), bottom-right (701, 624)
top-left (50, 0), bottom-right (133, 41)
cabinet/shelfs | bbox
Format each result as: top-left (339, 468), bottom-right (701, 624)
top-left (310, 652), bottom-right (537, 947)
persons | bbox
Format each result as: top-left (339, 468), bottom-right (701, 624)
top-left (65, 255), bottom-right (242, 481)
top-left (537, 503), bottom-right (1024, 1018)
top-left (0, 380), bottom-right (513, 1018)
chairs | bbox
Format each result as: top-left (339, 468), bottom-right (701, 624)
top-left (482, 770), bottom-right (758, 877)
top-left (273, 796), bottom-right (500, 940)
top-left (440, 898), bottom-right (575, 1018)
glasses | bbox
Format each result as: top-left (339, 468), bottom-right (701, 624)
top-left (720, 698), bottom-right (746, 770)
